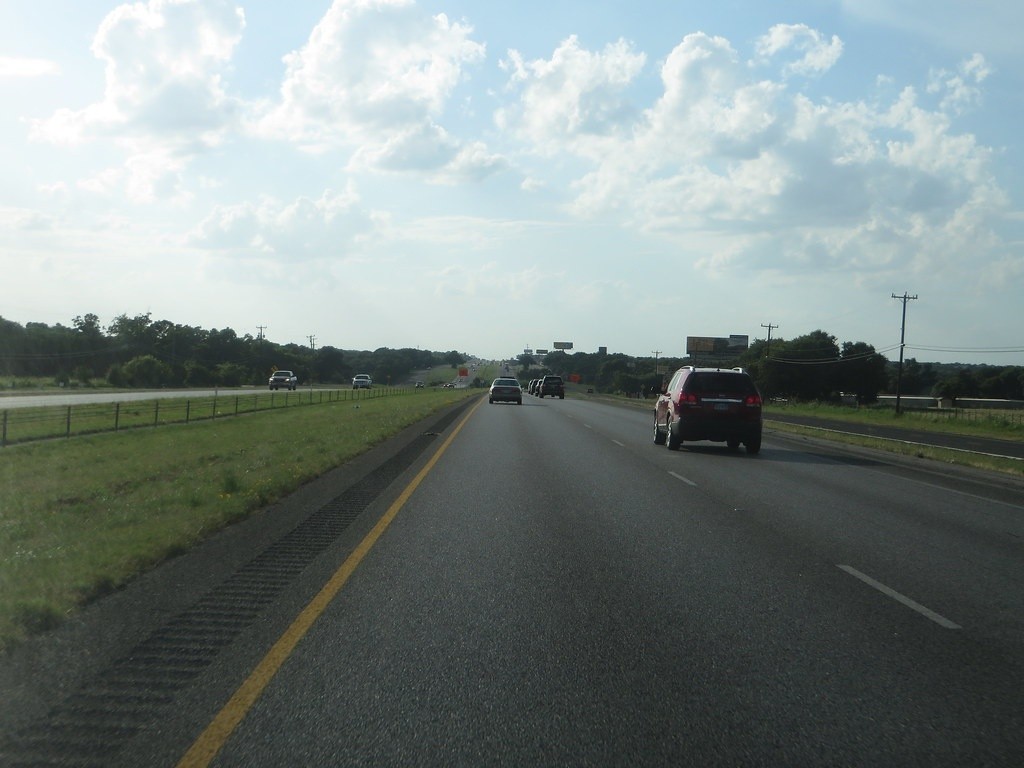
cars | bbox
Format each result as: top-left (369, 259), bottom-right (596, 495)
top-left (530, 379), bottom-right (539, 395)
top-left (489, 376), bottom-right (524, 405)
top-left (534, 379), bottom-right (543, 396)
top-left (415, 380), bottom-right (424, 388)
top-left (269, 370), bottom-right (297, 390)
top-left (528, 379), bottom-right (533, 394)
top-left (443, 381), bottom-right (457, 389)
top-left (352, 373), bottom-right (373, 390)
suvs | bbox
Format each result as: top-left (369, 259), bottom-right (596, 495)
top-left (539, 375), bottom-right (565, 399)
top-left (652, 365), bottom-right (765, 451)
